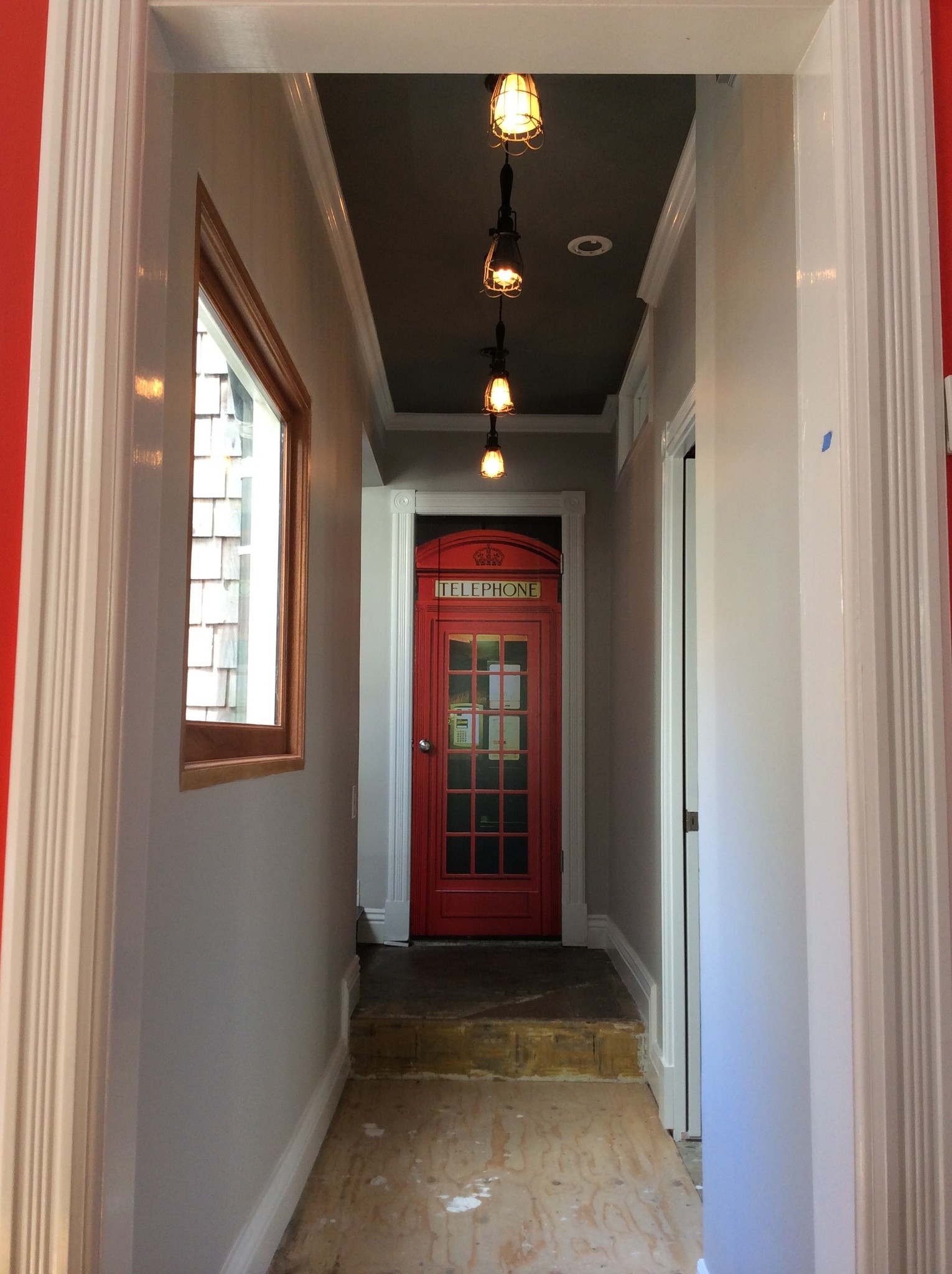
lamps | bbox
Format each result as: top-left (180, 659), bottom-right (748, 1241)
top-left (483, 73), bottom-right (545, 158)
top-left (480, 293), bottom-right (516, 418)
top-left (479, 140), bottom-right (524, 298)
top-left (478, 411), bottom-right (509, 480)
